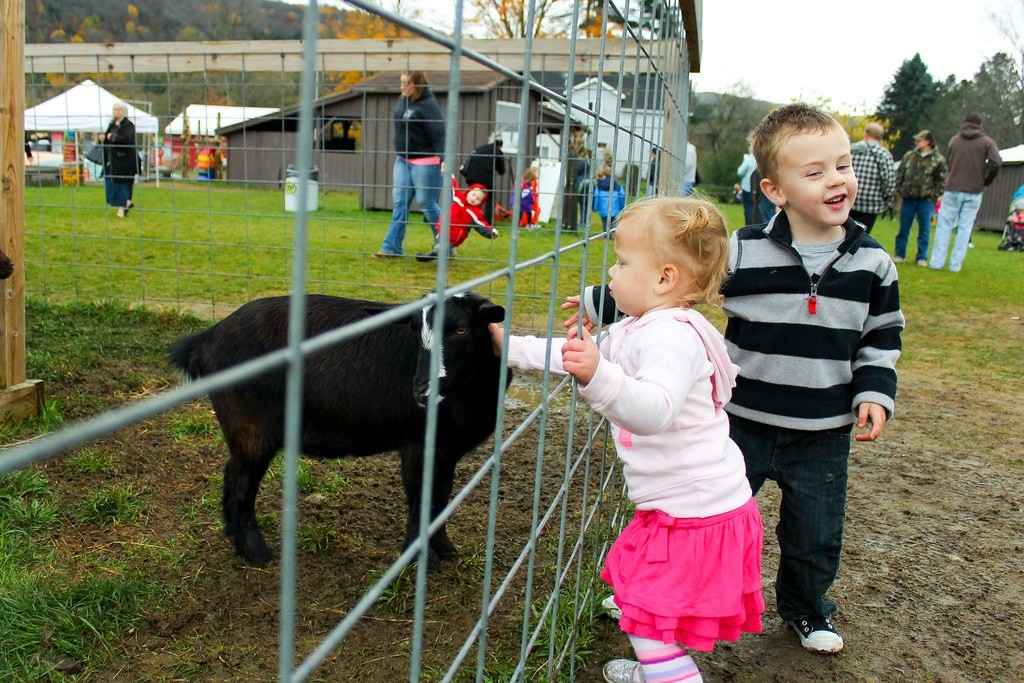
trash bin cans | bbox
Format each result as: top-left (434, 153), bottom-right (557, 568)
top-left (149, 166), bottom-right (171, 179)
top-left (284, 163), bottom-right (319, 212)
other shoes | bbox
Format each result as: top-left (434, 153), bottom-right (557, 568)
top-left (968, 242), bottom-right (974, 249)
top-left (526, 223), bottom-right (534, 230)
top-left (893, 256), bottom-right (906, 263)
top-left (603, 659), bottom-right (640, 683)
top-left (601, 595), bottom-right (622, 619)
top-left (117, 203), bottom-right (135, 218)
top-left (917, 259), bottom-right (928, 267)
top-left (370, 250), bottom-right (388, 259)
top-left (416, 252), bottom-right (438, 262)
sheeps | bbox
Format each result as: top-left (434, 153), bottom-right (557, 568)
top-left (166, 290), bottom-right (513, 578)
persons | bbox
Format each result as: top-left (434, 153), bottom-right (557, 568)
top-left (459, 140), bottom-right (505, 229)
top-left (99, 103), bottom-right (138, 220)
top-left (684, 139), bottom-right (697, 197)
top-left (562, 102), bottom-right (905, 655)
top-left (928, 112), bottom-right (1003, 273)
top-left (489, 198), bottom-right (764, 683)
top-left (733, 138), bottom-right (781, 226)
top-left (932, 190), bottom-right (1024, 248)
top-left (569, 129), bottom-right (624, 239)
top-left (370, 71), bottom-right (458, 260)
top-left (416, 174), bottom-right (499, 263)
top-left (25, 136), bottom-right (33, 161)
top-left (647, 149), bottom-right (662, 197)
top-left (882, 207), bottom-right (895, 222)
top-left (846, 123), bottom-right (896, 235)
top-left (508, 168), bottom-right (542, 230)
top-left (893, 131), bottom-right (949, 267)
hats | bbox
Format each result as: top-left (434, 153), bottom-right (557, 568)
top-left (912, 130), bottom-right (934, 142)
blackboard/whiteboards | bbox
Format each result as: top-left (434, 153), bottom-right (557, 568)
top-left (530, 159), bottom-right (566, 222)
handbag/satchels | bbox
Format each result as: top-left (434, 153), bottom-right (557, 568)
top-left (460, 150), bottom-right (476, 176)
top-left (85, 141), bottom-right (103, 166)
top-left (882, 206), bottom-right (895, 220)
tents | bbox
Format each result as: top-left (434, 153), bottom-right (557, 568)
top-left (24, 79), bottom-right (159, 191)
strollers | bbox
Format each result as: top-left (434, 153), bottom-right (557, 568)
top-left (998, 182), bottom-right (1024, 251)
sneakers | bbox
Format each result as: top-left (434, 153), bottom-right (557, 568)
top-left (788, 615), bottom-right (844, 654)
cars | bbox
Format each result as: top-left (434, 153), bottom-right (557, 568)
top-left (25, 139), bottom-right (104, 181)
